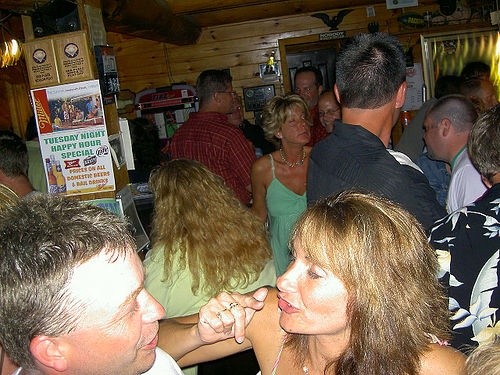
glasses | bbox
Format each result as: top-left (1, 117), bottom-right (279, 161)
top-left (212, 90), bottom-right (237, 99)
top-left (318, 107), bottom-right (341, 117)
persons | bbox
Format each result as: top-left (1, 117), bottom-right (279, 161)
top-left (0, 116), bottom-right (48, 210)
top-left (395, 61), bottom-right (498, 215)
top-left (426, 103), bottom-right (500, 355)
top-left (294, 66), bottom-right (331, 146)
top-left (319, 89), bottom-right (342, 134)
top-left (54, 95), bottom-right (103, 127)
top-left (141, 157), bottom-right (277, 375)
top-left (246, 92), bottom-right (312, 277)
top-left (455, 342), bottom-right (500, 375)
top-left (0, 194), bottom-right (185, 375)
top-left (167, 69), bottom-right (256, 208)
top-left (226, 89), bottom-right (279, 155)
top-left (156, 192), bottom-right (467, 375)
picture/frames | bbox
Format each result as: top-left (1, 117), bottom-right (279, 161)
top-left (243, 84), bottom-right (276, 112)
top-left (419, 26), bottom-right (500, 102)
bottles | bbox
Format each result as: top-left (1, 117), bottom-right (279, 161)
top-left (46, 154), bottom-right (68, 197)
top-left (266, 50), bottom-right (276, 74)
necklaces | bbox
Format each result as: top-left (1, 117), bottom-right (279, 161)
top-left (280, 147), bottom-right (306, 167)
top-left (301, 365), bottom-right (311, 375)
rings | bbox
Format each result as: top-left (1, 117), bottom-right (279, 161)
top-left (227, 302), bottom-right (239, 311)
top-left (216, 308), bottom-right (227, 317)
